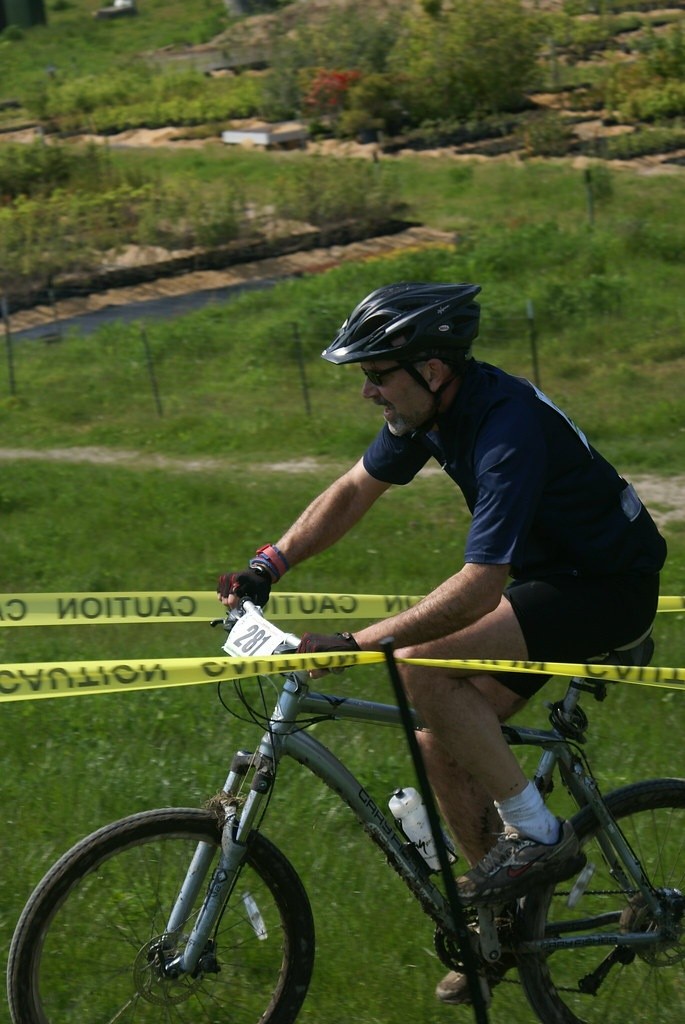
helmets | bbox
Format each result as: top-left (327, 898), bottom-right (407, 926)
top-left (321, 280), bottom-right (482, 365)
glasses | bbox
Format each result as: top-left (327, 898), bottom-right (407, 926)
top-left (360, 356), bottom-right (448, 387)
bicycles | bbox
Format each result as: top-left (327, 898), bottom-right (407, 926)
top-left (4, 586), bottom-right (685, 1024)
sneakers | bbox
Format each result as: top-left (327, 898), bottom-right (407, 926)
top-left (434, 901), bottom-right (521, 1006)
top-left (455, 816), bottom-right (587, 899)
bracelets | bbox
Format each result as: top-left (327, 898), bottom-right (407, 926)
top-left (249, 543), bottom-right (290, 584)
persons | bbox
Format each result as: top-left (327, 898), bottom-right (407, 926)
top-left (217, 283), bottom-right (669, 1005)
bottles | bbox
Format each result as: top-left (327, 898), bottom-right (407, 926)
top-left (388, 787), bottom-right (456, 871)
